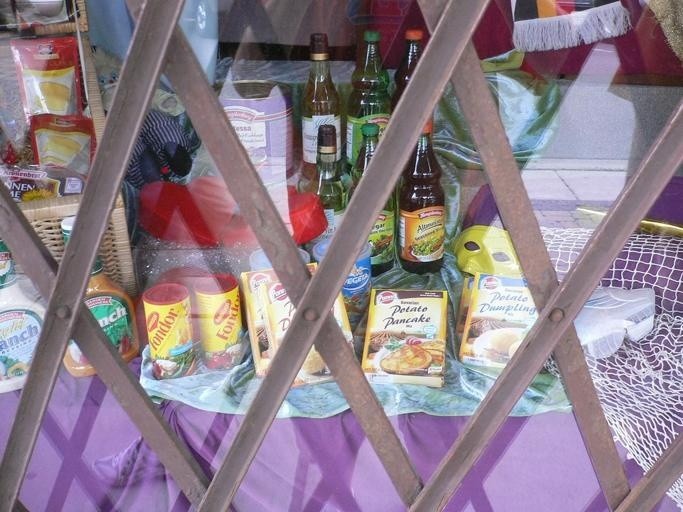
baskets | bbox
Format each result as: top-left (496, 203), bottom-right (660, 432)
top-left (0, 0), bottom-right (89, 37)
top-left (0, 1), bottom-right (140, 301)
top-left (0, 35), bottom-right (140, 301)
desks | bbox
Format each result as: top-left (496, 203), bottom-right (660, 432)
top-left (0, 57), bottom-right (683, 512)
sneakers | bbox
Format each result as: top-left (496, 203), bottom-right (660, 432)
top-left (92, 437), bottom-right (164, 486)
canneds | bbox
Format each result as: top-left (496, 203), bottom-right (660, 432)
top-left (313, 237), bottom-right (373, 335)
top-left (248, 246), bottom-right (310, 273)
top-left (141, 266), bottom-right (243, 382)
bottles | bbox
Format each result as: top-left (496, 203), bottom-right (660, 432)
top-left (51, 216), bottom-right (140, 379)
top-left (0, 237), bottom-right (49, 397)
top-left (296, 29), bottom-right (448, 289)
